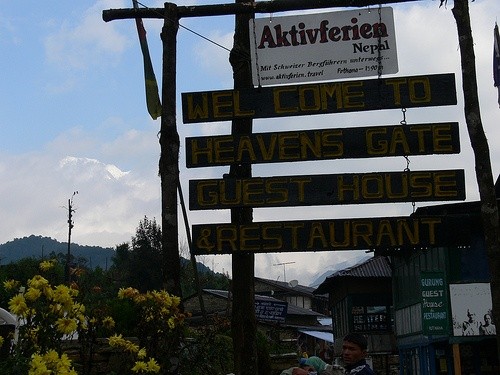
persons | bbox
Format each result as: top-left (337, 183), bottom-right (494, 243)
top-left (299, 345), bottom-right (334, 375)
top-left (463, 308), bottom-right (496, 336)
top-left (292, 331), bottom-right (374, 375)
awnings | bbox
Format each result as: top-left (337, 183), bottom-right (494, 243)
top-left (297, 329), bottom-right (334, 343)
